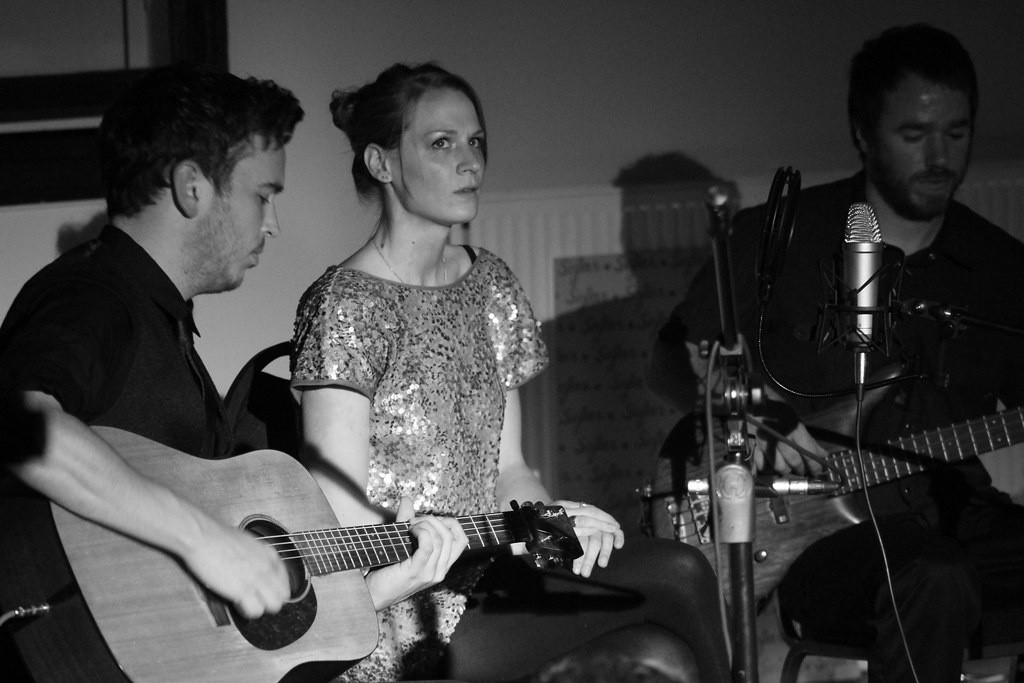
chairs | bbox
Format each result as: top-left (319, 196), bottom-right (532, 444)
top-left (772, 578), bottom-right (1024, 683)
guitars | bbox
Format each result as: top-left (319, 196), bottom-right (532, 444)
top-left (1, 422), bottom-right (588, 683)
top-left (645, 362), bottom-right (1024, 622)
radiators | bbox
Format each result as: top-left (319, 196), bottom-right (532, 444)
top-left (448, 157), bottom-right (1024, 472)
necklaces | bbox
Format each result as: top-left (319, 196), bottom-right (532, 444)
top-left (372, 238), bottom-right (447, 300)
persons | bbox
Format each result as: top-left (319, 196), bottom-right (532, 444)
top-left (291, 65), bottom-right (731, 683)
top-left (656, 24), bottom-right (1024, 683)
top-left (0, 62), bottom-right (469, 683)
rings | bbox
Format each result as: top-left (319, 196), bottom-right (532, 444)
top-left (580, 502), bottom-right (586, 509)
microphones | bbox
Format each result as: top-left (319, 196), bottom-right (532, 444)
top-left (842, 204), bottom-right (884, 400)
top-left (689, 476), bottom-right (841, 495)
